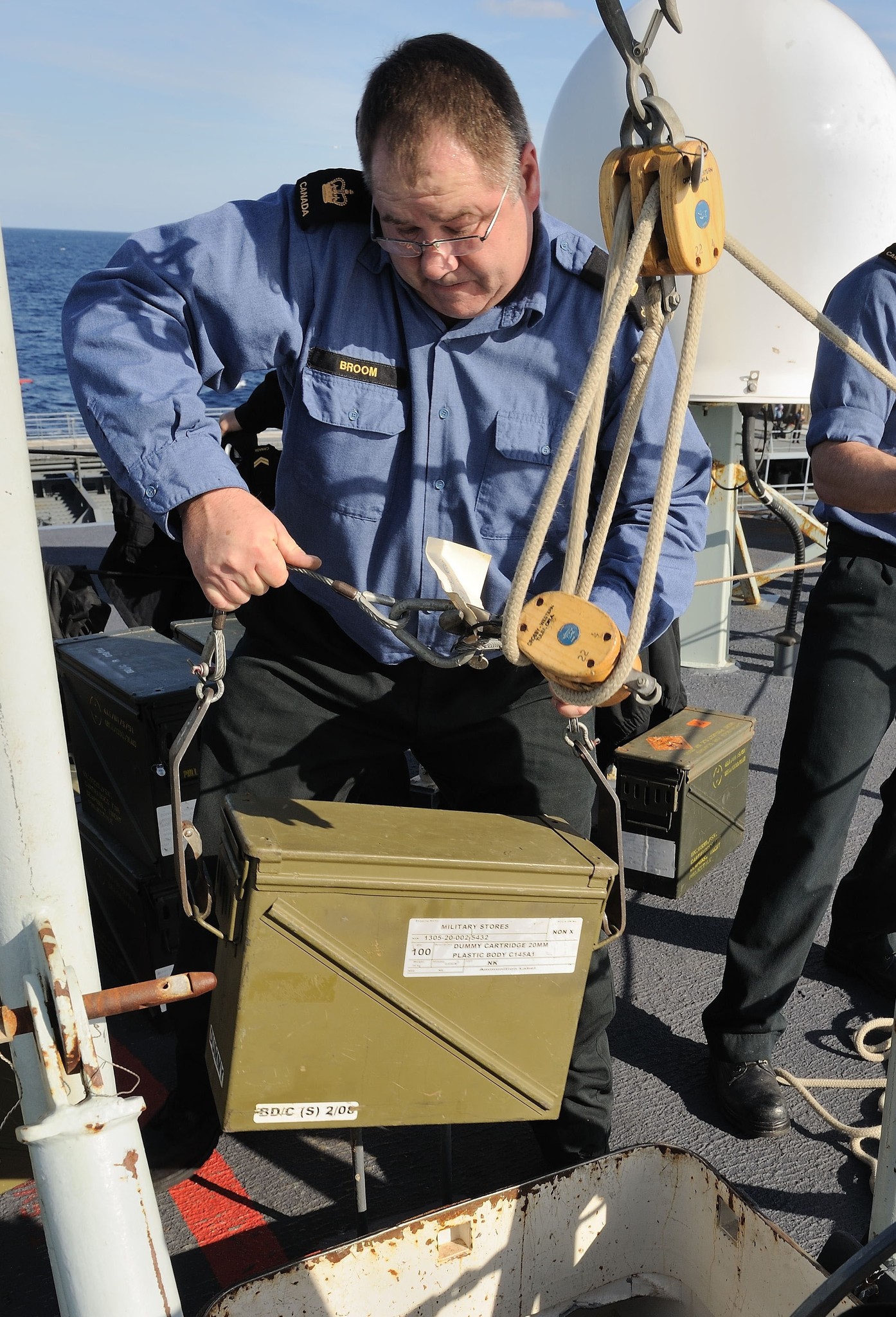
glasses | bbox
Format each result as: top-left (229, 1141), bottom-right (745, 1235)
top-left (370, 167), bottom-right (512, 258)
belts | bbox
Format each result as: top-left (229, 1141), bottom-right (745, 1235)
top-left (829, 522), bottom-right (896, 557)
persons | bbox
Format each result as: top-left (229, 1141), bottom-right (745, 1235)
top-left (61, 30), bottom-right (714, 1198)
top-left (699, 242), bottom-right (896, 1136)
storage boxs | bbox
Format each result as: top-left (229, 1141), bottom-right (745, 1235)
top-left (611, 705), bottom-right (758, 901)
top-left (203, 806), bottom-right (610, 1134)
top-left (73, 799), bottom-right (221, 1168)
top-left (49, 624), bottom-right (223, 876)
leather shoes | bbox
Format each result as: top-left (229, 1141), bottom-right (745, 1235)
top-left (823, 941), bottom-right (896, 1004)
top-left (710, 1050), bottom-right (791, 1138)
